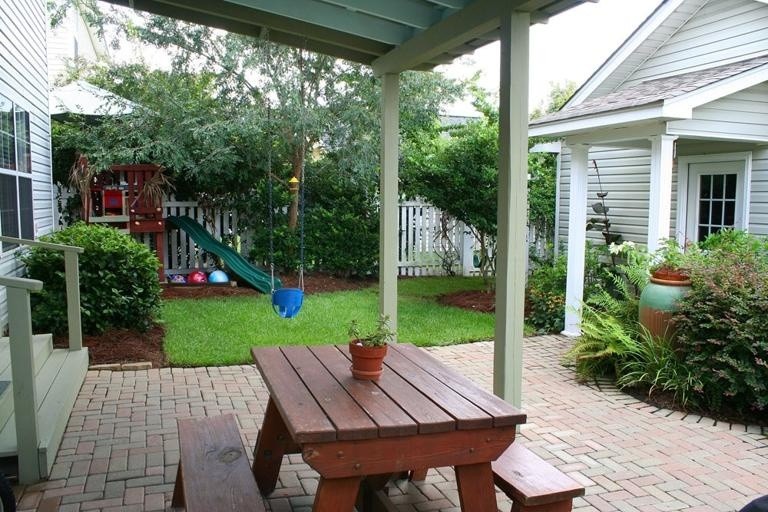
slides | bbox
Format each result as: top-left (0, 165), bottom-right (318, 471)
top-left (165, 215), bottom-right (281, 294)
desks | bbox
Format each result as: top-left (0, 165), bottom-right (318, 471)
top-left (250, 342), bottom-right (527, 512)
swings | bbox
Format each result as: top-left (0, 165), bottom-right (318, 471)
top-left (265, 29), bottom-right (306, 318)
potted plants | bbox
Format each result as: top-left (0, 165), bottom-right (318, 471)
top-left (344, 311), bottom-right (395, 380)
top-left (639, 235), bottom-right (689, 281)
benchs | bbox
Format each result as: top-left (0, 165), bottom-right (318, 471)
top-left (491, 441), bottom-right (586, 512)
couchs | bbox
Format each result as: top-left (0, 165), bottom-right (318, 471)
top-left (171, 413), bottom-right (270, 512)
